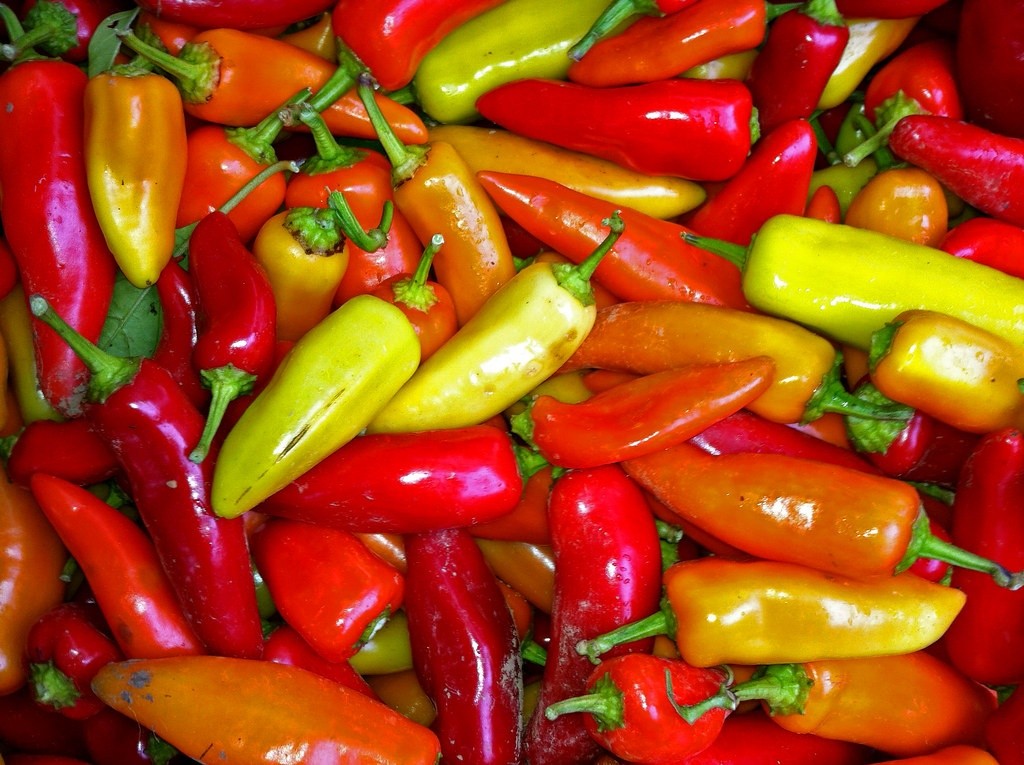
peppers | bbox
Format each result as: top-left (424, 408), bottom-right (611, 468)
top-left (0, 0), bottom-right (1024, 765)
